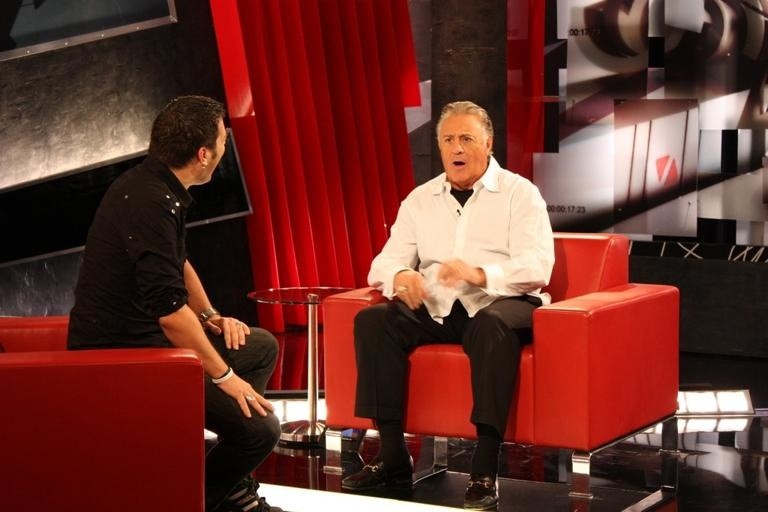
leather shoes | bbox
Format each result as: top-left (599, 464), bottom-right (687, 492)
top-left (460, 473), bottom-right (501, 510)
top-left (340, 452), bottom-right (414, 489)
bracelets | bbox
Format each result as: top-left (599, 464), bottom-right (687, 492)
top-left (199, 308), bottom-right (220, 326)
top-left (212, 367), bottom-right (234, 384)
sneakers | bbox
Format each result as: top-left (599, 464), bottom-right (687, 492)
top-left (225, 475), bottom-right (287, 511)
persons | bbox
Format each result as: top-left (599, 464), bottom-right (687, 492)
top-left (341, 101), bottom-right (555, 510)
top-left (66, 96), bottom-right (284, 512)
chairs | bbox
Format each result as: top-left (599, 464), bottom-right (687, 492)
top-left (324, 232), bottom-right (680, 498)
top-left (0, 316), bottom-right (205, 511)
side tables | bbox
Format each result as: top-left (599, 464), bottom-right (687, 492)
top-left (248, 288), bottom-right (355, 443)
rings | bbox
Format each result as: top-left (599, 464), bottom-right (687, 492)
top-left (236, 322), bottom-right (244, 327)
top-left (398, 285), bottom-right (407, 293)
top-left (245, 394), bottom-right (255, 400)
